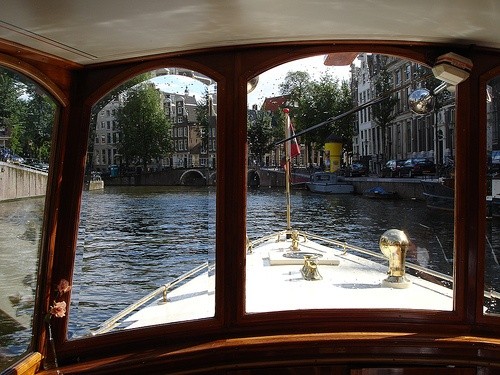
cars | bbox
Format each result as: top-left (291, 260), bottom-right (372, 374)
top-left (399, 159), bottom-right (435, 177)
top-left (379, 160), bottom-right (406, 177)
top-left (343, 164), bottom-right (368, 176)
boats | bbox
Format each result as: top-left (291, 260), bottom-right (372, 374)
top-left (364, 186), bottom-right (399, 201)
top-left (308, 170), bottom-right (355, 194)
